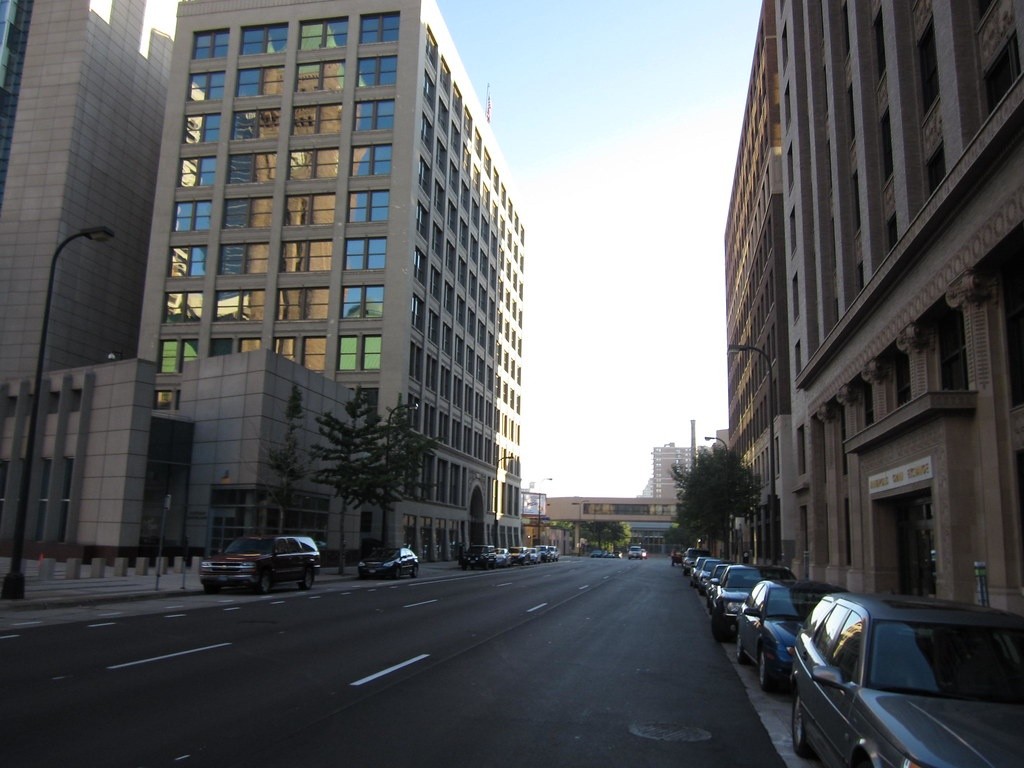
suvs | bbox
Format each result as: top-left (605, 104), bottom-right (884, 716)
top-left (200, 534), bottom-right (321, 596)
top-left (680, 547), bottom-right (712, 574)
top-left (791, 592), bottom-right (1023, 767)
top-left (626, 545), bottom-right (642, 560)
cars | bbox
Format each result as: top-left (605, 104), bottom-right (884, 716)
top-left (641, 549), bottom-right (647, 559)
top-left (735, 580), bottom-right (850, 693)
top-left (590, 549), bottom-right (623, 559)
top-left (457, 543), bottom-right (558, 570)
top-left (710, 563), bottom-right (798, 642)
top-left (696, 559), bottom-right (727, 597)
top-left (704, 563), bottom-right (729, 609)
top-left (689, 557), bottom-right (713, 589)
top-left (358, 547), bottom-right (419, 581)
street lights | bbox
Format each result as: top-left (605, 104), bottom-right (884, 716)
top-left (727, 344), bottom-right (781, 565)
top-left (0, 226), bottom-right (114, 600)
top-left (494, 457), bottom-right (516, 545)
top-left (704, 436), bottom-right (734, 561)
top-left (538, 478), bottom-right (552, 544)
top-left (381, 402), bottom-right (419, 549)
